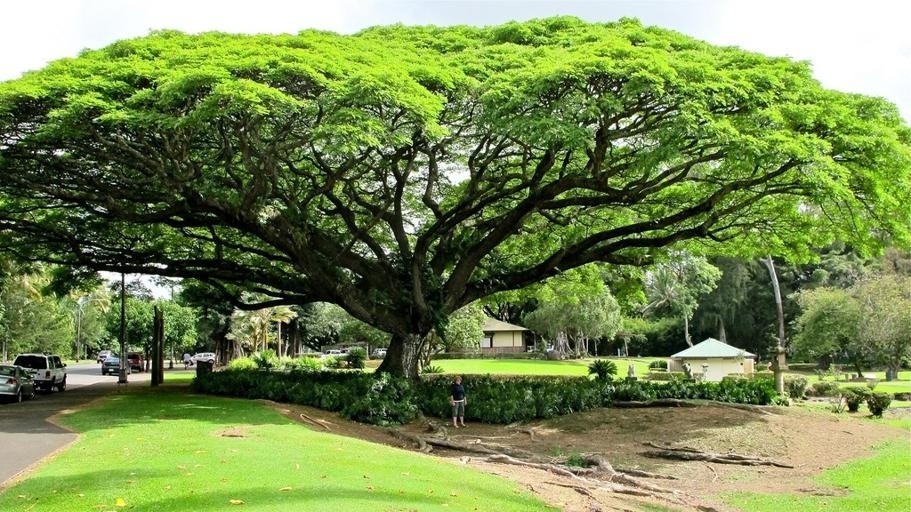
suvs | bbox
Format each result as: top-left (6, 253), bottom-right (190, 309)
top-left (14, 352), bottom-right (67, 392)
top-left (127, 353), bottom-right (144, 371)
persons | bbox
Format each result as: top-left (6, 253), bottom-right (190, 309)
top-left (182, 350), bottom-right (191, 370)
top-left (449, 374), bottom-right (470, 428)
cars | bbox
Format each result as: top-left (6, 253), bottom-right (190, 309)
top-left (102, 356), bottom-right (132, 375)
top-left (373, 348), bottom-right (388, 358)
top-left (0, 365), bottom-right (36, 402)
top-left (191, 352), bottom-right (216, 363)
top-left (323, 350), bottom-right (342, 356)
top-left (97, 350), bottom-right (112, 363)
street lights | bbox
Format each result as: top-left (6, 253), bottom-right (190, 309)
top-left (111, 281), bottom-right (127, 380)
top-left (73, 299), bottom-right (92, 361)
